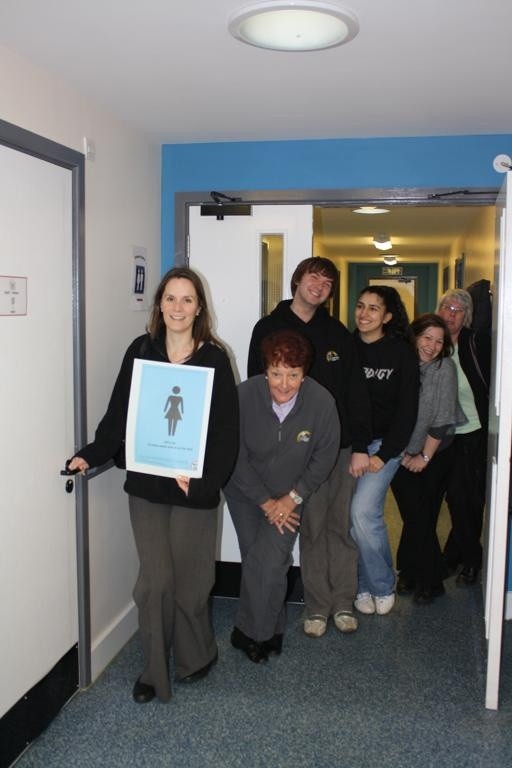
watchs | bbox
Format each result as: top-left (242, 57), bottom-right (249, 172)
top-left (288, 490), bottom-right (304, 506)
top-left (421, 450), bottom-right (431, 468)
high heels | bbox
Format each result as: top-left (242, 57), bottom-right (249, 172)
top-left (230, 626), bottom-right (254, 650)
top-left (261, 633), bottom-right (283, 659)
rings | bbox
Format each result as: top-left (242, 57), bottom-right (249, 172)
top-left (279, 513), bottom-right (284, 518)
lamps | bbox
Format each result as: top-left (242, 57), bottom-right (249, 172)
top-left (374, 237), bottom-right (393, 251)
top-left (352, 206), bottom-right (390, 215)
top-left (226, 2), bottom-right (358, 54)
top-left (384, 256), bottom-right (397, 265)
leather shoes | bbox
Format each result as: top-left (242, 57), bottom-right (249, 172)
top-left (304, 613), bottom-right (327, 638)
top-left (133, 676), bottom-right (156, 703)
top-left (332, 608), bottom-right (359, 635)
top-left (414, 578), bottom-right (448, 608)
top-left (394, 569), bottom-right (414, 594)
top-left (187, 652), bottom-right (219, 678)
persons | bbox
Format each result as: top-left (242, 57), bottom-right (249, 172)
top-left (223, 331), bottom-right (341, 666)
top-left (246, 256), bottom-right (372, 639)
top-left (398, 314), bottom-right (458, 607)
top-left (69, 266), bottom-right (242, 704)
top-left (340, 285), bottom-right (419, 617)
top-left (434, 288), bottom-right (491, 588)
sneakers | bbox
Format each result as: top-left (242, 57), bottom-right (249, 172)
top-left (375, 594), bottom-right (395, 616)
top-left (352, 592), bottom-right (375, 613)
